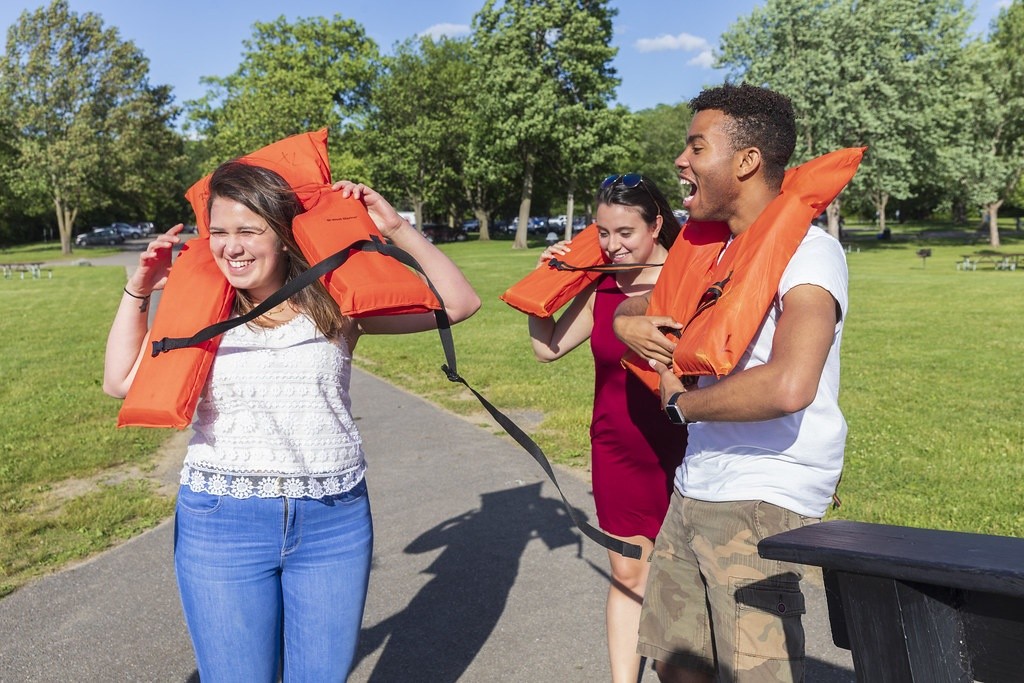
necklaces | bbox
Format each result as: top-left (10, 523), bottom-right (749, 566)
top-left (267, 307), bottom-right (286, 316)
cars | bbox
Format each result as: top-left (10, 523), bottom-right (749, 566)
top-left (185, 222), bottom-right (199, 235)
top-left (410, 224), bottom-right (470, 245)
top-left (673, 209), bottom-right (691, 224)
top-left (461, 214), bottom-right (598, 235)
top-left (812, 213), bottom-right (828, 227)
top-left (74, 222), bottom-right (155, 248)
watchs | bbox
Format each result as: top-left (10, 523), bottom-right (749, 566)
top-left (664, 392), bottom-right (686, 425)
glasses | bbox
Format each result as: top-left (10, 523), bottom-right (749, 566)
top-left (602, 172), bottom-right (660, 216)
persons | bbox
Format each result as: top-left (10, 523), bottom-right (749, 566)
top-left (529, 174), bottom-right (698, 683)
top-left (612, 83), bottom-right (851, 683)
top-left (102, 160), bottom-right (483, 683)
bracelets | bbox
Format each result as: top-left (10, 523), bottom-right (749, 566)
top-left (124, 286), bottom-right (151, 312)
